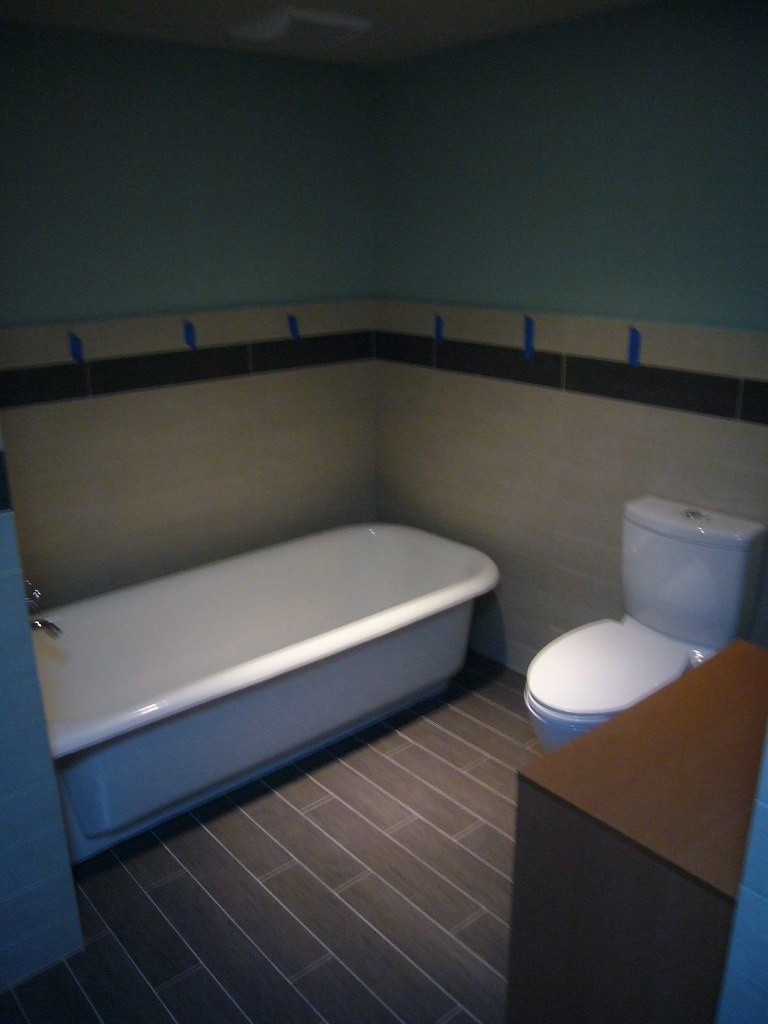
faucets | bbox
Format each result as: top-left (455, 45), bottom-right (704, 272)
top-left (25, 578), bottom-right (62, 639)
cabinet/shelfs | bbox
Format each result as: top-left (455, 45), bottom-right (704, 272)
top-left (505, 638), bottom-right (768, 1024)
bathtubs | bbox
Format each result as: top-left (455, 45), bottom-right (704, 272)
top-left (35, 522), bottom-right (504, 869)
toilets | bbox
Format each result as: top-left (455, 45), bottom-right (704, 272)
top-left (522, 495), bottom-right (768, 760)
